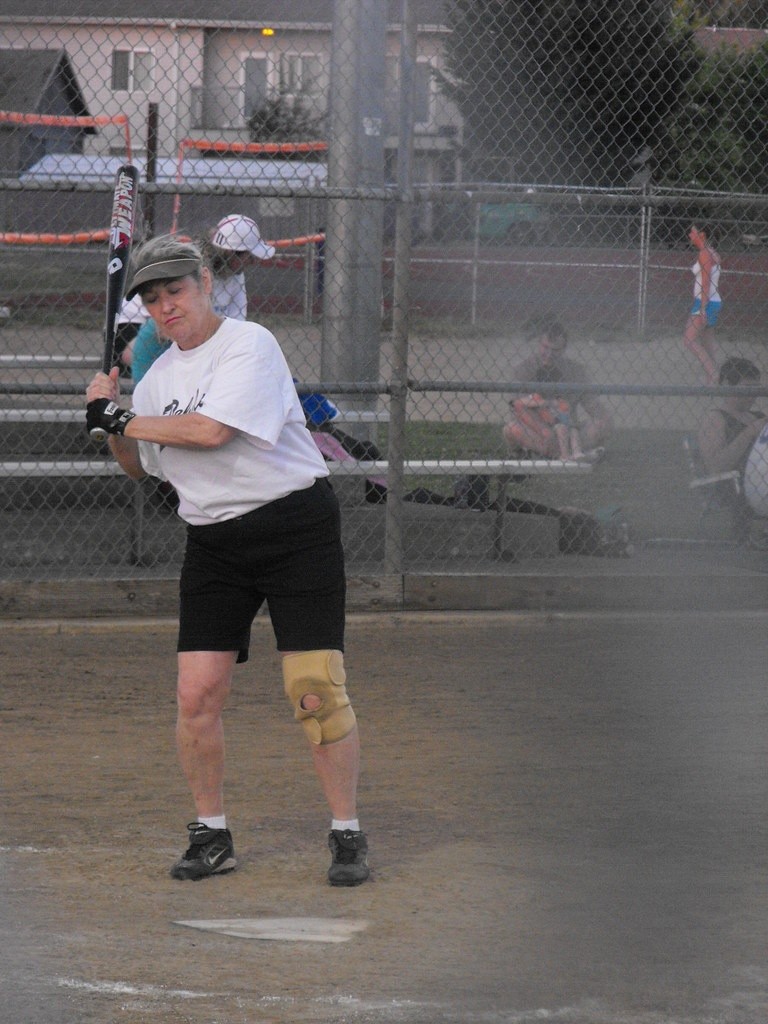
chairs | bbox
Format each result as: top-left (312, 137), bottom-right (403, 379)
top-left (683, 438), bottom-right (768, 550)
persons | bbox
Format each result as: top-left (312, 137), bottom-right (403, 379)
top-left (496, 322), bottom-right (615, 516)
top-left (85, 214), bottom-right (370, 886)
top-left (684, 221), bottom-right (723, 390)
top-left (699, 359), bottom-right (768, 546)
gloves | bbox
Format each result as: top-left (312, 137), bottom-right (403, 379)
top-left (85, 398), bottom-right (137, 437)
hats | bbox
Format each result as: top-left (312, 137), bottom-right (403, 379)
top-left (211, 214), bottom-right (276, 260)
top-left (125, 236), bottom-right (201, 302)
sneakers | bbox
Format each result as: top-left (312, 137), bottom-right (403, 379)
top-left (168, 822), bottom-right (238, 881)
top-left (326, 828), bottom-right (369, 887)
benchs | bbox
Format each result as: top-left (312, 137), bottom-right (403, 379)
top-left (0, 459), bottom-right (595, 565)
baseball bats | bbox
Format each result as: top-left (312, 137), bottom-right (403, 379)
top-left (89, 167), bottom-right (138, 441)
